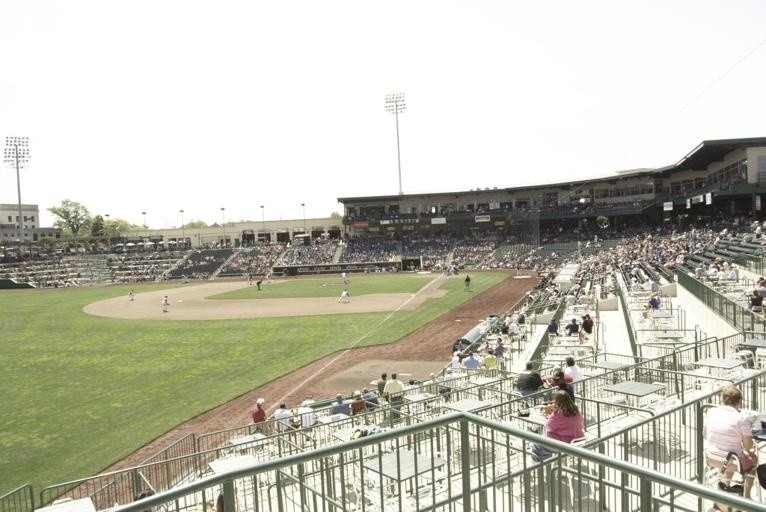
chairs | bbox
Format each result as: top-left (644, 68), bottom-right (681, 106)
top-left (440, 288), bottom-right (762, 512)
top-left (99, 379), bottom-right (448, 512)
top-left (0, 219), bottom-right (766, 288)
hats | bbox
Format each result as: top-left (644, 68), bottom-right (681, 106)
top-left (257, 398), bottom-right (264, 406)
top-left (551, 370), bottom-right (564, 381)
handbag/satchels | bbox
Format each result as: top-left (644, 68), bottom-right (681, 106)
top-left (714, 479), bottom-right (744, 512)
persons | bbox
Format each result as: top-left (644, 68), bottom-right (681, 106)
top-left (162, 296), bottom-right (170, 312)
top-left (581, 316), bottom-right (592, 335)
top-left (444, 230), bottom-right (576, 278)
top-left (445, 370), bottom-right (456, 388)
top-left (534, 276), bottom-right (589, 309)
top-left (493, 338), bottom-right (507, 356)
top-left (406, 380), bottom-right (418, 395)
top-left (114, 240), bottom-right (224, 285)
top-left (351, 391), bottom-right (364, 416)
top-left (547, 368), bottom-right (574, 388)
top-left (644, 295), bottom-right (658, 310)
top-left (544, 369), bottom-right (576, 405)
top-left (301, 395), bottom-right (314, 406)
top-left (651, 278), bottom-right (660, 292)
top-left (378, 373), bottom-right (388, 396)
top-left (530, 389), bottom-right (585, 463)
top-left (464, 275), bottom-right (470, 291)
top-left (222, 238), bottom-right (282, 273)
top-left (383, 372), bottom-right (405, 420)
top-left (247, 273), bottom-right (254, 286)
top-left (516, 361), bottom-right (543, 399)
top-left (280, 232), bottom-right (338, 272)
top-left (703, 385), bottom-right (757, 502)
top-left (484, 351), bottom-right (498, 369)
top-left (343, 230), bottom-right (451, 273)
top-left (548, 319), bottom-right (559, 336)
top-left (505, 314), bottom-right (513, 324)
top-left (1, 242), bottom-right (100, 288)
top-left (746, 276), bottom-right (766, 311)
top-left (363, 388), bottom-right (378, 412)
top-left (586, 314), bottom-right (593, 327)
top-left (332, 394), bottom-right (350, 416)
top-left (344, 208), bottom-right (450, 223)
top-left (479, 342), bottom-right (492, 356)
top-left (464, 351), bottom-right (480, 369)
top-left (509, 320), bottom-right (520, 333)
top-left (517, 313), bottom-right (526, 323)
top-left (128, 290), bottom-right (134, 301)
top-left (501, 322), bottom-right (510, 334)
top-left (275, 402), bottom-right (294, 431)
top-left (644, 278), bottom-right (652, 291)
top-left (257, 278), bottom-right (262, 291)
top-left (338, 289), bottom-right (351, 303)
top-left (297, 402), bottom-right (316, 428)
top-left (252, 399), bottom-right (267, 423)
top-left (632, 279), bottom-right (641, 291)
top-left (452, 351), bottom-right (462, 368)
top-left (424, 373), bottom-right (440, 394)
top-left (573, 214), bottom-right (766, 279)
top-left (565, 318), bottom-right (578, 336)
top-left (565, 357), bottom-right (584, 390)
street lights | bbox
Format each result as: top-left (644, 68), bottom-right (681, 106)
top-left (141, 212), bottom-right (146, 236)
top-left (4, 137), bottom-right (31, 251)
top-left (105, 214), bottom-right (110, 243)
top-left (180, 210), bottom-right (184, 236)
top-left (302, 204), bottom-right (305, 231)
top-left (260, 206), bottom-right (264, 230)
top-left (385, 93), bottom-right (407, 194)
top-left (220, 208), bottom-right (225, 236)
